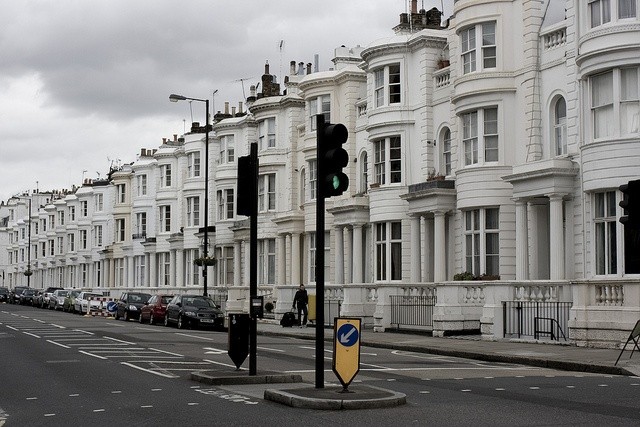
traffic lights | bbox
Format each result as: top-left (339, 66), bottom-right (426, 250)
top-left (318, 122), bottom-right (350, 198)
top-left (235, 158), bottom-right (256, 217)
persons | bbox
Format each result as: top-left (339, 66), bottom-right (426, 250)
top-left (293, 284), bottom-right (308, 328)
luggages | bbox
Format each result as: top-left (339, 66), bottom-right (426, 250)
top-left (280, 307), bottom-right (296, 327)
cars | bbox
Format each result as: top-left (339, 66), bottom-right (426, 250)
top-left (164, 294), bottom-right (225, 329)
top-left (20, 289), bottom-right (39, 305)
top-left (31, 291), bottom-right (48, 306)
top-left (47, 290), bottom-right (68, 309)
top-left (0, 287), bottom-right (10, 302)
top-left (74, 292), bottom-right (103, 312)
top-left (138, 294), bottom-right (174, 323)
top-left (115, 292), bottom-right (152, 320)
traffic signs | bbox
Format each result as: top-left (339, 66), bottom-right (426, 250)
top-left (331, 314), bottom-right (363, 388)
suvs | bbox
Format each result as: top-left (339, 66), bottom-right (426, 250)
top-left (66, 289), bottom-right (81, 310)
top-left (41, 288), bottom-right (64, 308)
top-left (11, 287), bottom-right (29, 304)
